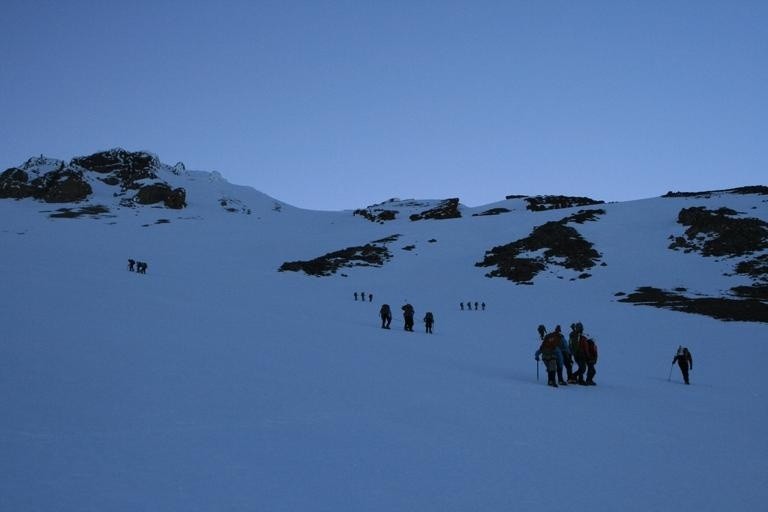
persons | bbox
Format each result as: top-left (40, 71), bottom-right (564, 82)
top-left (140, 262), bottom-right (147, 275)
top-left (534, 322), bottom-right (599, 386)
top-left (136, 261), bottom-right (141, 273)
top-left (474, 302), bottom-right (478, 310)
top-left (127, 258), bottom-right (136, 272)
top-left (354, 292), bottom-right (358, 301)
top-left (672, 344), bottom-right (693, 385)
top-left (378, 303), bottom-right (392, 329)
top-left (402, 303), bottom-right (416, 332)
top-left (467, 302), bottom-right (472, 310)
top-left (423, 312), bottom-right (434, 334)
top-left (482, 302), bottom-right (485, 310)
top-left (369, 294), bottom-right (373, 302)
top-left (460, 302), bottom-right (464, 310)
top-left (361, 292), bottom-right (365, 301)
